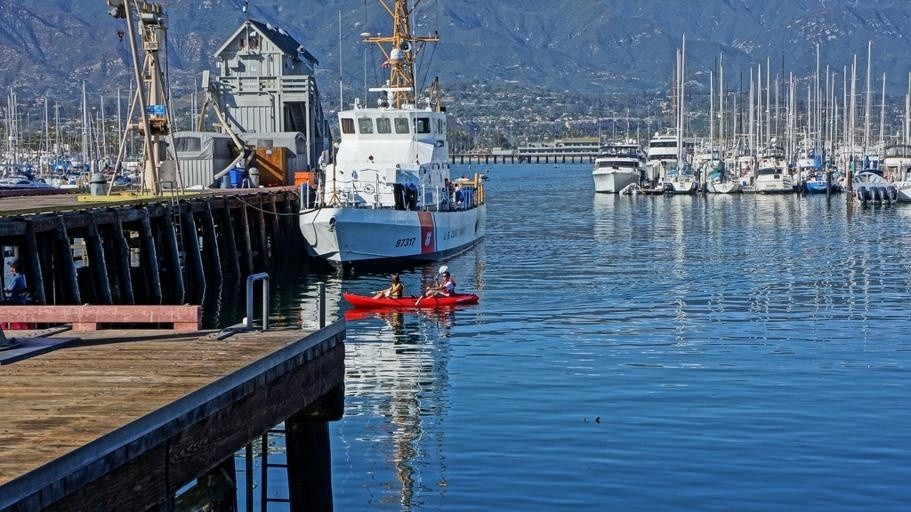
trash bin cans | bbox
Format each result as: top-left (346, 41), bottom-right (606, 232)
top-left (91, 173), bottom-right (106, 195)
top-left (230, 167), bottom-right (247, 188)
top-left (249, 167), bottom-right (260, 188)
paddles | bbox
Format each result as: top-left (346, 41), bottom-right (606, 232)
top-left (415, 265), bottom-right (447, 306)
top-left (369, 289), bottom-right (386, 295)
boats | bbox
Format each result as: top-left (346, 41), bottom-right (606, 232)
top-left (298, 1), bottom-right (487, 274)
top-left (341, 291), bottom-right (478, 306)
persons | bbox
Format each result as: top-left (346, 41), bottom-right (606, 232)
top-left (419, 308), bottom-right (456, 339)
top-left (423, 269), bottom-right (456, 299)
top-left (3, 261), bottom-right (27, 298)
top-left (373, 272), bottom-right (404, 299)
top-left (373, 311), bottom-right (405, 345)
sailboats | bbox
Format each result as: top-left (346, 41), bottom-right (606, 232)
top-left (0, 80), bottom-right (200, 189)
